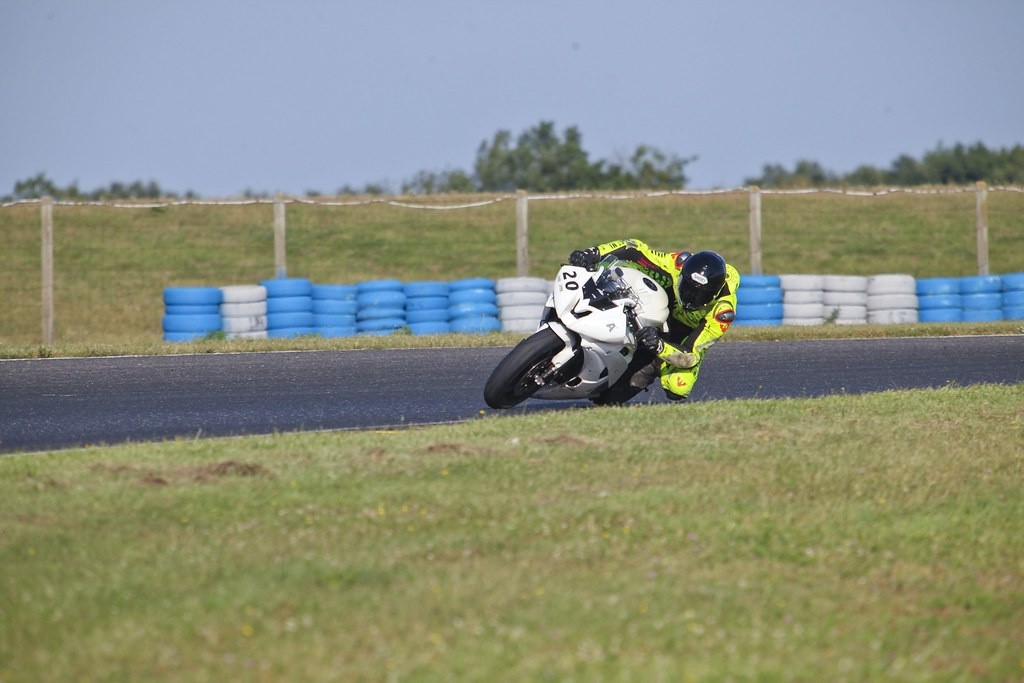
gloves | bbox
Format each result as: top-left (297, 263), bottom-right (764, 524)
top-left (569, 247), bottom-right (599, 268)
top-left (636, 326), bottom-right (664, 354)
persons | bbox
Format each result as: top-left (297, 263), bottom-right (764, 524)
top-left (570, 238), bottom-right (740, 402)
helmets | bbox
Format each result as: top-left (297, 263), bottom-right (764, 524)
top-left (679, 251), bottom-right (726, 310)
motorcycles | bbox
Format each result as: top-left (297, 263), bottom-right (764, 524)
top-left (484, 254), bottom-right (671, 412)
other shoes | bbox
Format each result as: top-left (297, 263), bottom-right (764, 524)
top-left (629, 360), bottom-right (662, 391)
